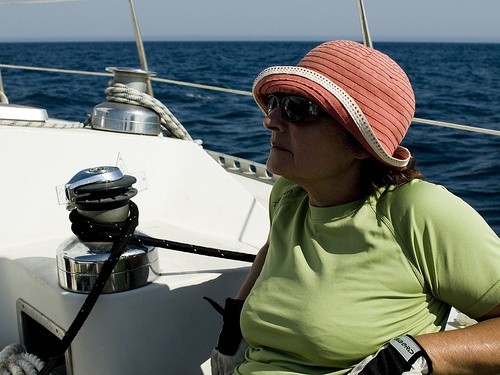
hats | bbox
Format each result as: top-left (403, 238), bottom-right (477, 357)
top-left (253, 39), bottom-right (415, 171)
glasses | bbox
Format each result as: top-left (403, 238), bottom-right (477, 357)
top-left (268, 94), bottom-right (332, 127)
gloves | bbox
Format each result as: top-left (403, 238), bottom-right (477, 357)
top-left (211, 298), bottom-right (243, 374)
top-left (347, 334), bottom-right (433, 375)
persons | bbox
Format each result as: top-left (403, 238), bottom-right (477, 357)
top-left (211, 40), bottom-right (500, 375)
